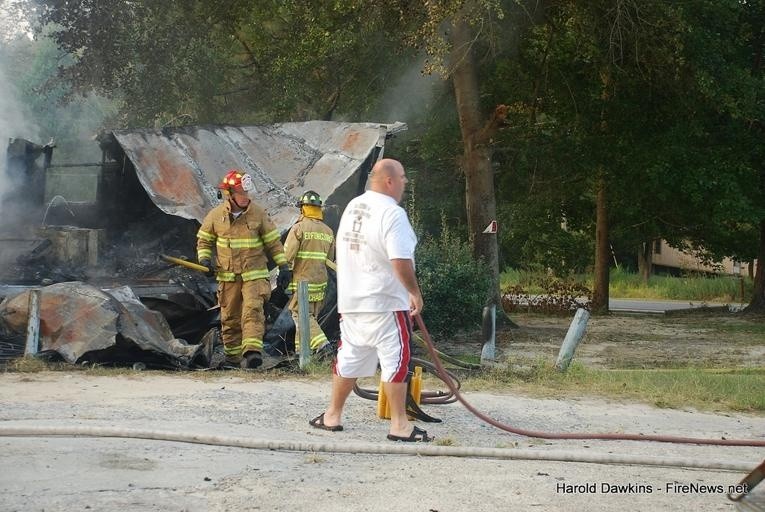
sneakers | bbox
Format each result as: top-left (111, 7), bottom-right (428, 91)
top-left (316, 344), bottom-right (334, 361)
top-left (216, 351), bottom-right (263, 369)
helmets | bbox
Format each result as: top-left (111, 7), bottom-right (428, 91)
top-left (217, 170), bottom-right (252, 191)
top-left (295, 190), bottom-right (322, 207)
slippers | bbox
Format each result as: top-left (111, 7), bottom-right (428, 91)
top-left (387, 425), bottom-right (436, 442)
top-left (309, 412), bottom-right (343, 431)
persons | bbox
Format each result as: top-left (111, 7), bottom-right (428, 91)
top-left (284, 190), bottom-right (335, 362)
top-left (197, 171), bottom-right (290, 369)
top-left (309, 159), bottom-right (436, 441)
